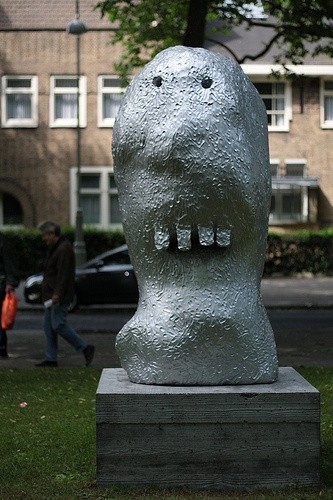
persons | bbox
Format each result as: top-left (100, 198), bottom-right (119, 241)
top-left (37, 220), bottom-right (95, 367)
top-left (0, 231), bottom-right (21, 359)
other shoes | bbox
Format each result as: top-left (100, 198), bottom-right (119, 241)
top-left (82, 344), bottom-right (96, 366)
top-left (34, 360), bottom-right (59, 367)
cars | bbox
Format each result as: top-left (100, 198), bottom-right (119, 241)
top-left (22, 245), bottom-right (142, 306)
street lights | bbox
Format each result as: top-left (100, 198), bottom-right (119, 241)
top-left (66, 15), bottom-right (88, 268)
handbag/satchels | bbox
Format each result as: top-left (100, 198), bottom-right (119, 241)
top-left (1, 291), bottom-right (17, 329)
